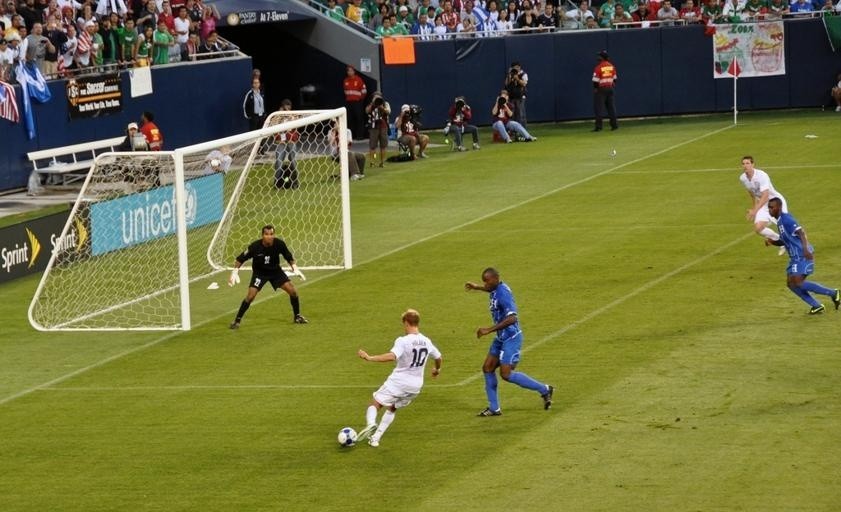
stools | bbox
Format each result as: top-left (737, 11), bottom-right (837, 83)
top-left (493, 129), bottom-right (511, 143)
top-left (448, 134), bottom-right (464, 151)
top-left (398, 141), bottom-right (410, 155)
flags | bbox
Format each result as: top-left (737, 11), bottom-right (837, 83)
top-left (0, 80), bottom-right (20, 125)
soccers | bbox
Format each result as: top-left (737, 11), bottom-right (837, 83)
top-left (337, 426), bottom-right (357, 448)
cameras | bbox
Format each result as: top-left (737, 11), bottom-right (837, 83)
top-left (405, 104), bottom-right (424, 127)
top-left (373, 97), bottom-right (384, 106)
top-left (456, 100), bottom-right (464, 109)
top-left (498, 97), bottom-right (506, 105)
top-left (510, 69), bottom-right (518, 76)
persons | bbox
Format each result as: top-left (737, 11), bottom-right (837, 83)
top-left (464, 268), bottom-right (554, 416)
top-left (448, 96), bottom-right (481, 152)
top-left (831, 82), bottom-right (841, 112)
top-left (327, 126), bottom-right (366, 180)
top-left (326, 0), bottom-right (841, 41)
top-left (591, 50), bottom-right (618, 131)
top-left (739, 156), bottom-right (788, 256)
top-left (242, 78), bottom-right (267, 155)
top-left (505, 61), bottom-right (528, 127)
top-left (395, 105), bottom-right (429, 160)
top-left (272, 100), bottom-right (299, 190)
top-left (765, 200), bottom-right (841, 315)
top-left (120, 123), bottom-right (150, 154)
top-left (0, 0), bottom-right (227, 83)
top-left (227, 224), bottom-right (307, 329)
top-left (253, 69), bottom-right (261, 79)
top-left (491, 90), bottom-right (537, 143)
top-left (342, 64), bottom-right (366, 114)
top-left (355, 310), bottom-right (442, 447)
top-left (365, 92), bottom-right (391, 168)
top-left (139, 109), bottom-right (163, 152)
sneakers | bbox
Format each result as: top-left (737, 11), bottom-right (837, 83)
top-left (351, 175), bottom-right (360, 181)
top-left (230, 321), bottom-right (240, 329)
top-left (831, 289), bottom-right (841, 310)
top-left (506, 138), bottom-right (512, 143)
top-left (834, 105), bottom-right (841, 112)
top-left (478, 406), bottom-right (501, 417)
top-left (420, 152), bottom-right (430, 159)
top-left (472, 143), bottom-right (481, 150)
top-left (541, 384), bottom-right (553, 410)
top-left (458, 145), bottom-right (468, 152)
top-left (778, 245), bottom-right (786, 256)
top-left (529, 135), bottom-right (537, 142)
top-left (356, 423), bottom-right (379, 442)
top-left (367, 435), bottom-right (379, 447)
top-left (809, 303), bottom-right (825, 314)
top-left (359, 174), bottom-right (365, 179)
top-left (591, 127), bottom-right (601, 132)
top-left (294, 314), bottom-right (308, 324)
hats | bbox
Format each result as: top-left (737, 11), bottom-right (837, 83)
top-left (0, 39), bottom-right (7, 45)
top-left (7, 31), bottom-right (21, 42)
top-left (127, 122), bottom-right (138, 131)
top-left (596, 48), bottom-right (609, 58)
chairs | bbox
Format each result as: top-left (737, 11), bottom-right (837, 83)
top-left (26, 136), bottom-right (127, 185)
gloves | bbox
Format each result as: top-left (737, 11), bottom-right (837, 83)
top-left (293, 269), bottom-right (306, 281)
top-left (228, 271), bottom-right (241, 287)
